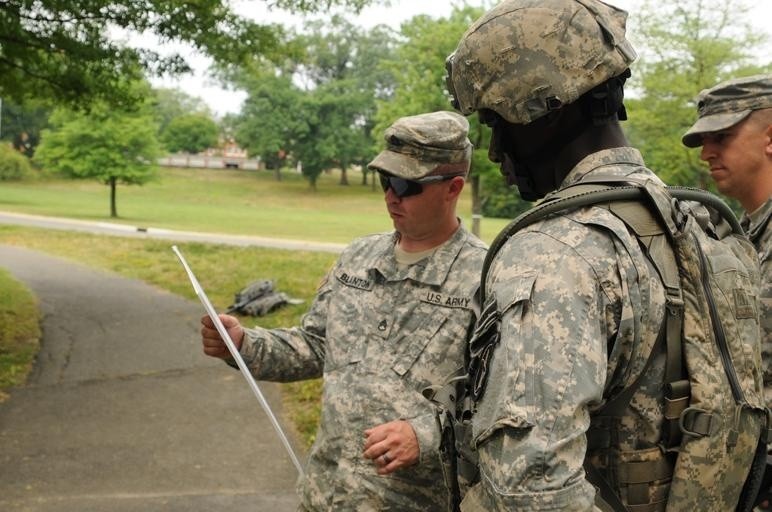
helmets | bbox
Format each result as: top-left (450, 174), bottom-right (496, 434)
top-left (446, 0), bottom-right (637, 128)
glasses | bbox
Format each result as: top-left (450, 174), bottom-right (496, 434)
top-left (377, 172), bottom-right (465, 197)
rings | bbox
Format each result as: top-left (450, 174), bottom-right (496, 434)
top-left (384, 454), bottom-right (392, 464)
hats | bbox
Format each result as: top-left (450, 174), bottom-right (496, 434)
top-left (367, 110), bottom-right (473, 181)
top-left (681, 74), bottom-right (772, 147)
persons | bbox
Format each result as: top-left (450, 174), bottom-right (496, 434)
top-left (682, 74), bottom-right (772, 466)
top-left (445, 0), bottom-right (772, 511)
top-left (201, 110), bottom-right (490, 512)
